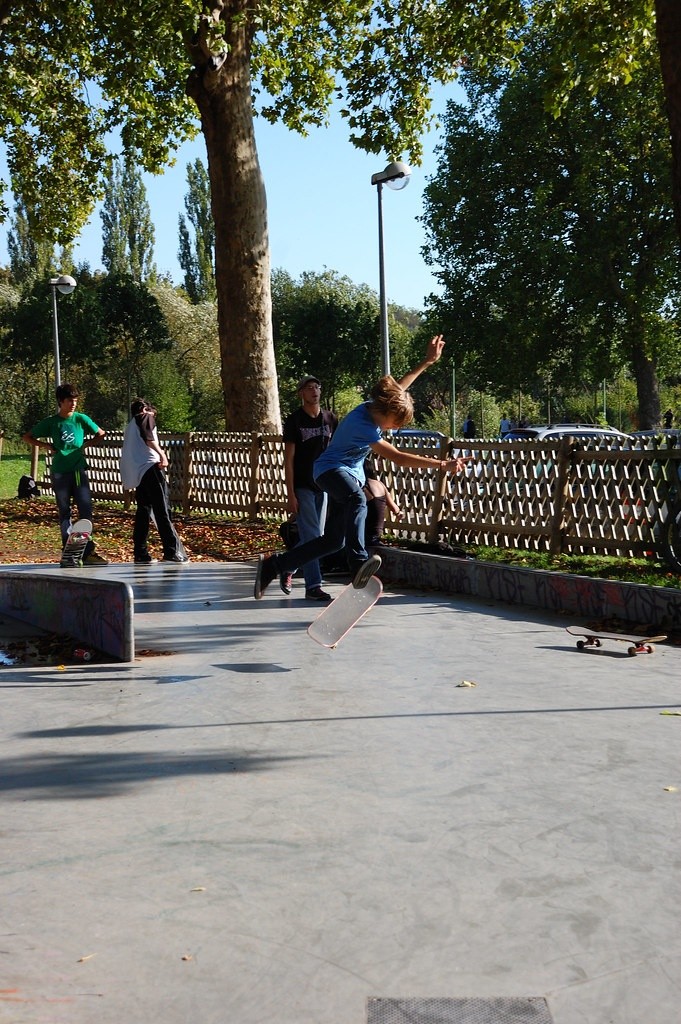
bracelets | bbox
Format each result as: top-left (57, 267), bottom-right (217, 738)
top-left (439, 460), bottom-right (443, 470)
top-left (393, 510), bottom-right (401, 515)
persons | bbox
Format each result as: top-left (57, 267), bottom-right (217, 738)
top-left (316, 458), bottom-right (403, 578)
top-left (663, 408), bottom-right (674, 429)
top-left (120, 399), bottom-right (190, 565)
top-left (278, 375), bottom-right (340, 600)
top-left (499, 414), bottom-right (528, 440)
top-left (23, 384), bottom-right (110, 565)
top-left (255, 335), bottom-right (476, 600)
top-left (462, 415), bottom-right (476, 440)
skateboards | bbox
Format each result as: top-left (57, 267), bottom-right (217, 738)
top-left (566, 626), bottom-right (668, 657)
top-left (134, 490), bottom-right (190, 564)
top-left (60, 518), bottom-right (92, 568)
top-left (307, 576), bottom-right (383, 650)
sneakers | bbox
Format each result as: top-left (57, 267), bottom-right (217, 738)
top-left (305, 588), bottom-right (330, 601)
top-left (254, 552), bottom-right (273, 600)
top-left (281, 571), bottom-right (294, 594)
top-left (352, 555), bottom-right (382, 589)
top-left (83, 549), bottom-right (108, 564)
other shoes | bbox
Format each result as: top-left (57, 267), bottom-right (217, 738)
top-left (134, 560), bottom-right (156, 566)
top-left (323, 564), bottom-right (351, 576)
top-left (280, 522), bottom-right (299, 551)
top-left (162, 555), bottom-right (190, 565)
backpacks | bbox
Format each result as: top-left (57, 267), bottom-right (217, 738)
top-left (467, 420), bottom-right (476, 436)
top-left (18, 475), bottom-right (40, 498)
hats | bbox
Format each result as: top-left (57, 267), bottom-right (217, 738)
top-left (501, 412), bottom-right (506, 415)
top-left (297, 376), bottom-right (321, 391)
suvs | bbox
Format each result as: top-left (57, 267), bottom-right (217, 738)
top-left (488, 424), bottom-right (681, 543)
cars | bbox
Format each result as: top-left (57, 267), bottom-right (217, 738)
top-left (377, 429), bottom-right (484, 508)
top-left (624, 429), bottom-right (681, 450)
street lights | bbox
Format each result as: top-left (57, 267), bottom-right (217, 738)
top-left (48, 275), bottom-right (77, 414)
top-left (371, 162), bottom-right (413, 440)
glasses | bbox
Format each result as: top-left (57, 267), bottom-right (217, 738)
top-left (306, 383), bottom-right (321, 391)
